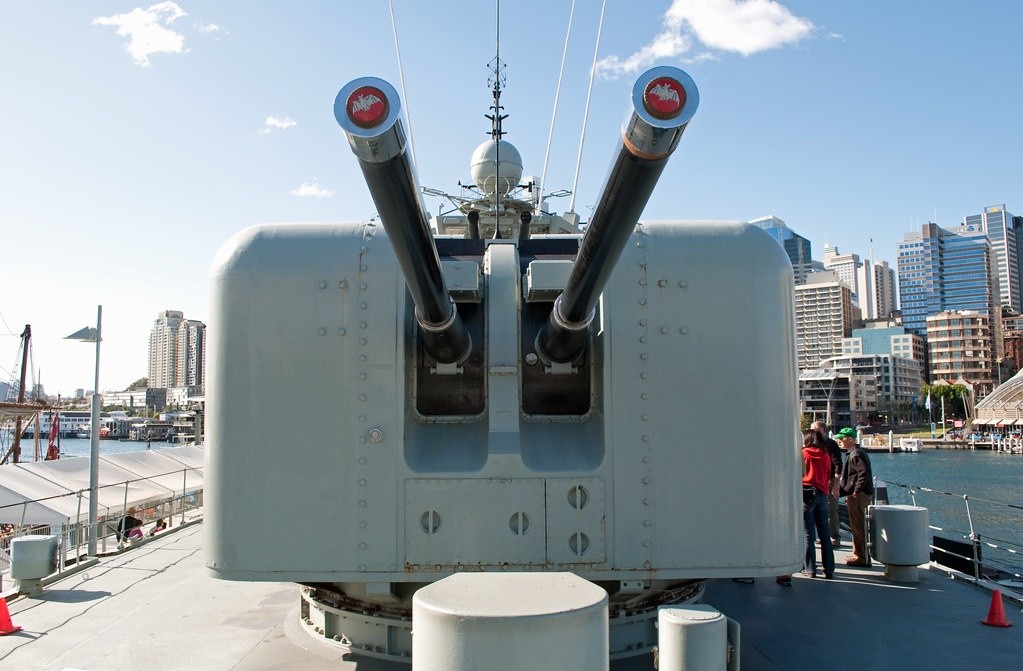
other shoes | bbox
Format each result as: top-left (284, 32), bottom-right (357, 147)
top-left (847, 559), bottom-right (871, 567)
top-left (824, 573), bottom-right (834, 578)
top-left (831, 539), bottom-right (840, 548)
top-left (810, 573), bottom-right (815, 578)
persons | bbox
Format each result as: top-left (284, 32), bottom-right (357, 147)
top-left (837, 428), bottom-right (873, 568)
top-left (129, 519), bottom-right (143, 542)
top-left (809, 421), bottom-right (843, 550)
top-left (802, 429), bottom-right (835, 579)
top-left (733, 574), bottom-right (793, 585)
top-left (116, 507), bottom-right (142, 541)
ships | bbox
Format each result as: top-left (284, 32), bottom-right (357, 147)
top-left (8, 410), bottom-right (117, 439)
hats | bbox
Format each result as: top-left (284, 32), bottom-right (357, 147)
top-left (834, 428), bottom-right (857, 439)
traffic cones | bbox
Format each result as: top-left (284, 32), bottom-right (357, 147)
top-left (981, 590), bottom-right (1014, 628)
top-left (0, 596), bottom-right (22, 636)
